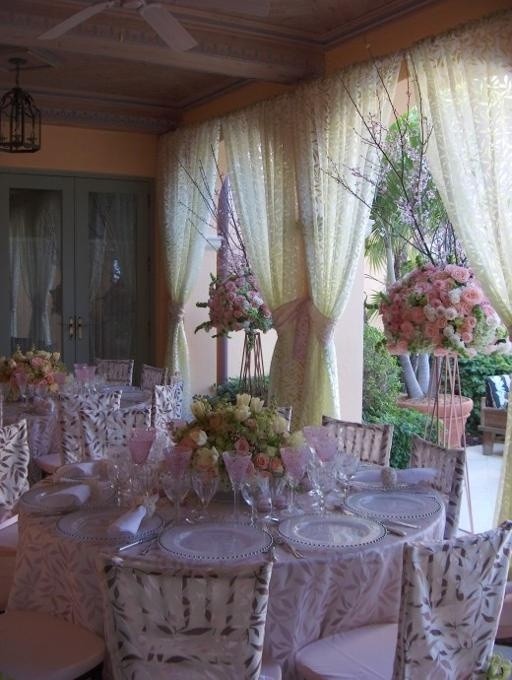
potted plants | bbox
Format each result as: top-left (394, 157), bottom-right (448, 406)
top-left (316, 46), bottom-right (474, 449)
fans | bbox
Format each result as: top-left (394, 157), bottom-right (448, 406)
top-left (35, 0), bottom-right (200, 55)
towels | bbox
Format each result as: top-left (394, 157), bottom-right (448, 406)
top-left (108, 492), bottom-right (160, 534)
top-left (46, 483), bottom-right (93, 506)
top-left (65, 460), bottom-right (95, 479)
top-left (351, 468), bottom-right (439, 484)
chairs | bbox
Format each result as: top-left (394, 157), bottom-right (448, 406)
top-left (409, 433), bottom-right (466, 541)
top-left (321, 415), bottom-right (394, 466)
top-left (292, 522), bottom-right (512, 680)
top-left (95, 554), bottom-right (269, 680)
top-left (77, 407), bottom-right (130, 457)
top-left (150, 381), bottom-right (183, 427)
top-left (0, 610), bottom-right (106, 680)
top-left (275, 405), bottom-right (294, 432)
top-left (479, 373), bottom-right (512, 455)
top-left (95, 358), bottom-right (131, 385)
top-left (37, 389), bottom-right (123, 478)
top-left (0, 418), bottom-right (30, 523)
top-left (140, 363), bottom-right (168, 389)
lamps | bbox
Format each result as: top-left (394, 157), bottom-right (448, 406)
top-left (0, 56), bottom-right (44, 150)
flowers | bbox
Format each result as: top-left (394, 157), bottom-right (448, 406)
top-left (165, 390), bottom-right (308, 479)
top-left (195, 268), bottom-right (274, 351)
top-left (377, 261), bottom-right (501, 360)
top-left (0, 342), bottom-right (69, 399)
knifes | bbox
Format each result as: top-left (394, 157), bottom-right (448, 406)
top-left (341, 506), bottom-right (408, 537)
top-left (116, 534), bottom-right (158, 552)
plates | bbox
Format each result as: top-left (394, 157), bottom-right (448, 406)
top-left (340, 465), bottom-right (409, 490)
top-left (158, 524), bottom-right (273, 561)
top-left (53, 461), bottom-right (102, 481)
top-left (56, 509), bottom-right (165, 542)
top-left (278, 514), bottom-right (387, 549)
top-left (343, 491), bottom-right (441, 520)
top-left (19, 483), bottom-right (100, 512)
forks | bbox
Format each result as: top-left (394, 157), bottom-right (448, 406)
top-left (270, 527), bottom-right (303, 559)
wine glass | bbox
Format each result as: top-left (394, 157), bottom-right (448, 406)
top-left (258, 478), bottom-right (288, 524)
top-left (129, 427), bottom-right (155, 495)
top-left (163, 447), bottom-right (192, 474)
top-left (16, 372), bottom-right (29, 408)
top-left (64, 361), bottom-right (107, 396)
top-left (186, 472), bottom-right (219, 524)
top-left (161, 473), bottom-right (192, 518)
top-left (304, 426), bottom-right (347, 515)
top-left (240, 475), bottom-right (269, 527)
top-left (222, 450), bottom-right (253, 523)
top-left (334, 445), bottom-right (361, 511)
top-left (22, 382), bottom-right (35, 410)
top-left (136, 464), bottom-right (160, 520)
top-left (304, 466), bottom-right (336, 514)
top-left (280, 447), bottom-right (310, 517)
top-left (106, 455), bottom-right (132, 506)
top-left (166, 421), bottom-right (187, 445)
top-left (37, 383), bottom-right (49, 401)
